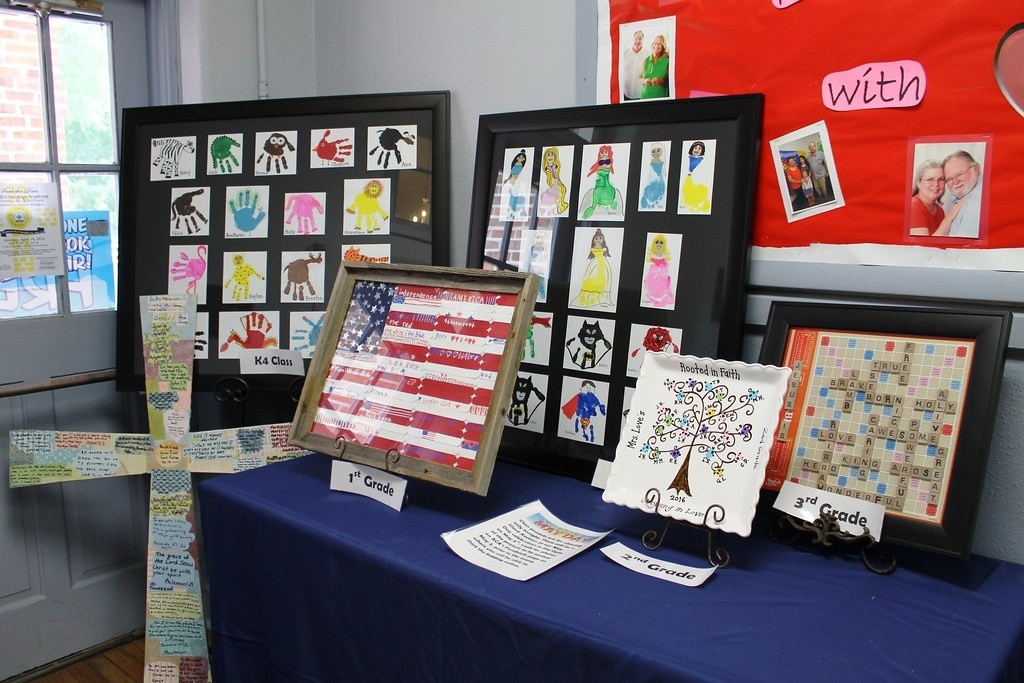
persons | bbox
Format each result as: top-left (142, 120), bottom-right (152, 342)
top-left (638, 35), bottom-right (669, 99)
top-left (787, 157), bottom-right (810, 208)
top-left (806, 141), bottom-right (830, 202)
top-left (623, 31), bottom-right (653, 100)
top-left (909, 159), bottom-right (966, 237)
top-left (801, 170), bottom-right (815, 205)
top-left (798, 155), bottom-right (812, 178)
top-left (942, 150), bottom-right (982, 239)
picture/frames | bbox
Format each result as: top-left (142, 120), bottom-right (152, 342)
top-left (115, 85), bottom-right (1013, 556)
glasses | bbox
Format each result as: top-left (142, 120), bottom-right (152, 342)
top-left (946, 164), bottom-right (981, 182)
top-left (916, 175), bottom-right (946, 184)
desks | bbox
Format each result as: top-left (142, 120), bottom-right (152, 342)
top-left (196, 453), bottom-right (1024, 683)
